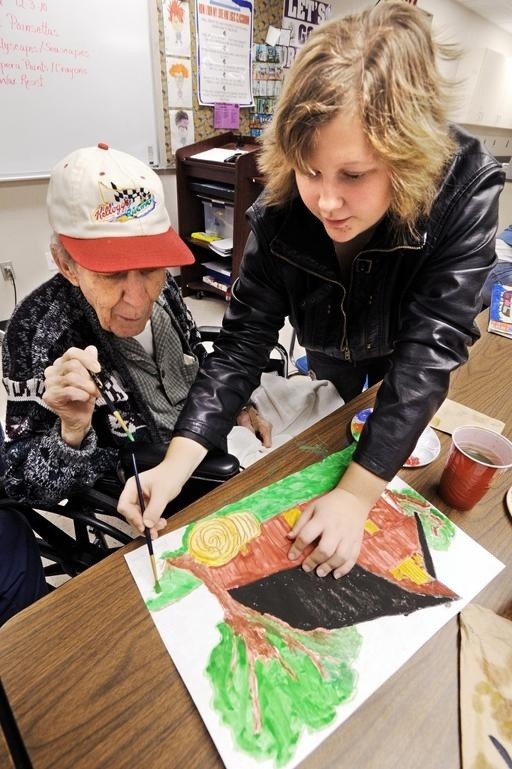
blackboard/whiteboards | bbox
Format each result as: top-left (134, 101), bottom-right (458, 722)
top-left (1, 0), bottom-right (166, 183)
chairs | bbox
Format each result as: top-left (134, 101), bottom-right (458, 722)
top-left (288, 328), bottom-right (368, 392)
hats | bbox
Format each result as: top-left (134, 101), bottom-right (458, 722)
top-left (45, 143), bottom-right (198, 271)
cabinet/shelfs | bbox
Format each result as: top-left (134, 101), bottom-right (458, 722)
top-left (175, 131), bottom-right (265, 308)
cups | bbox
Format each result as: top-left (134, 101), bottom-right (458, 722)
top-left (438, 423), bottom-right (511, 512)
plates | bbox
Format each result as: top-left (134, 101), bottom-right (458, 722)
top-left (348, 406), bottom-right (442, 469)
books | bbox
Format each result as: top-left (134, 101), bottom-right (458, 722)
top-left (199, 261), bottom-right (232, 277)
top-left (201, 274), bottom-right (231, 293)
top-left (190, 230), bottom-right (226, 244)
top-left (205, 269), bottom-right (232, 285)
top-left (208, 237), bottom-right (233, 259)
top-left (486, 280), bottom-right (512, 342)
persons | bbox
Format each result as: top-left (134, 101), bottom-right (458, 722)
top-left (2, 508), bottom-right (60, 630)
top-left (0, 140), bottom-right (276, 509)
top-left (115, 1), bottom-right (510, 585)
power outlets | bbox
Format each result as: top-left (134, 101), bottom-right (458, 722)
top-left (0, 261), bottom-right (16, 281)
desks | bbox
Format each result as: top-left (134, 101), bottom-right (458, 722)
top-left (1, 306), bottom-right (512, 769)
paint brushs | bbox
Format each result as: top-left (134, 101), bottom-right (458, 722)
top-left (131, 452), bottom-right (160, 586)
top-left (60, 339), bottom-right (135, 442)
